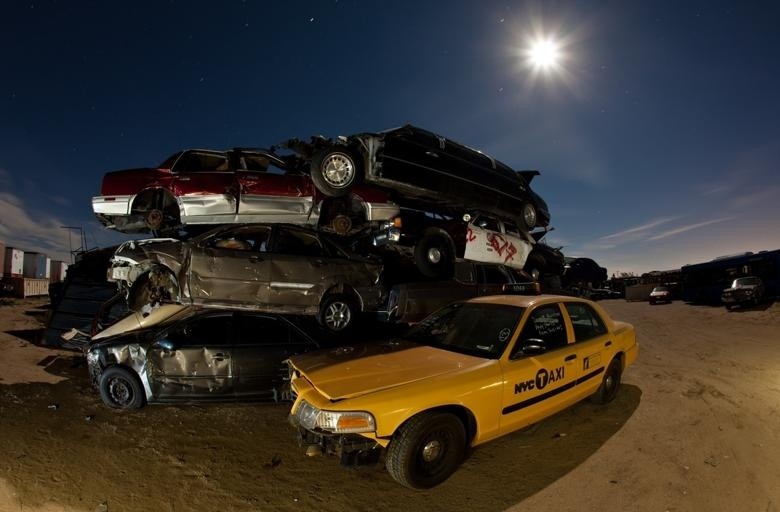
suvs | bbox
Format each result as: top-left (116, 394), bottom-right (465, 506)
top-left (720, 276), bottom-right (766, 310)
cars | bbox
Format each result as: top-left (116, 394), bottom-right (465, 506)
top-left (648, 286), bottom-right (672, 306)
